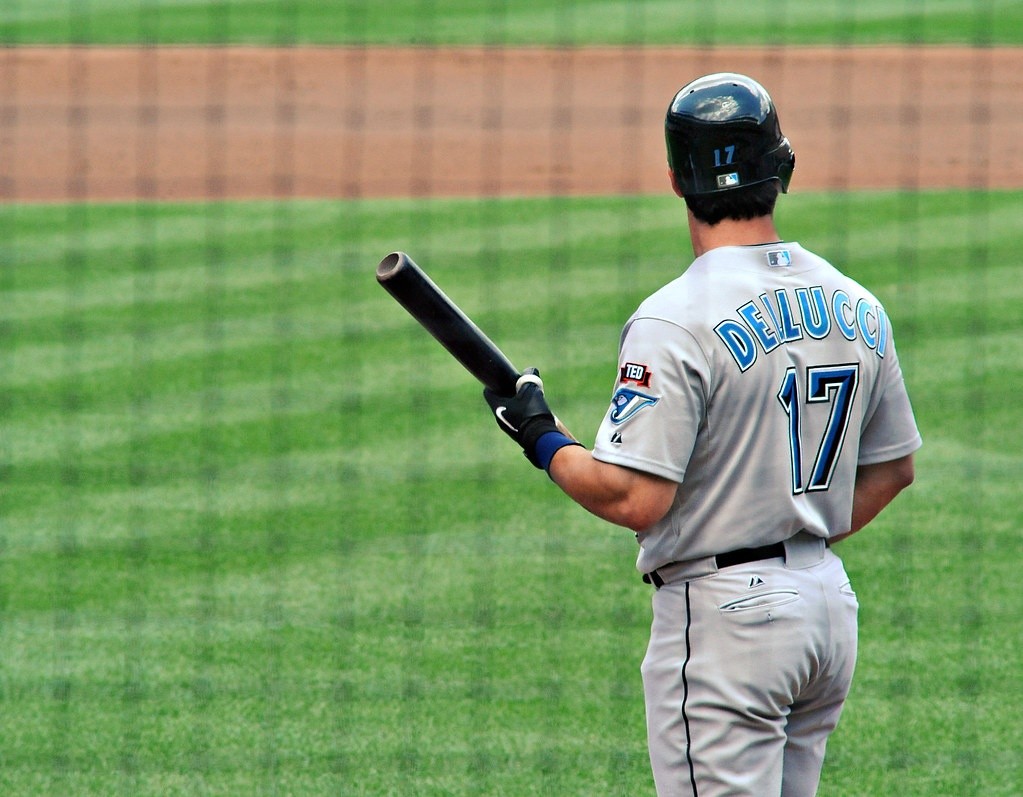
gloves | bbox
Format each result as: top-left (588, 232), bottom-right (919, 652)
top-left (483, 368), bottom-right (564, 470)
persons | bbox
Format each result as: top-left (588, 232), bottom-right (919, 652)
top-left (482, 74), bottom-right (922, 797)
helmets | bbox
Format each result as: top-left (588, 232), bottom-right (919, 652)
top-left (664, 73), bottom-right (795, 195)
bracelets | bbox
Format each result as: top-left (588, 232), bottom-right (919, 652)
top-left (536, 431), bottom-right (588, 480)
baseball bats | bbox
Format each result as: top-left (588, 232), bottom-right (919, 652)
top-left (375, 251), bottom-right (578, 442)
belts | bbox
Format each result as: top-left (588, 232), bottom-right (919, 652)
top-left (642, 537), bottom-right (831, 589)
